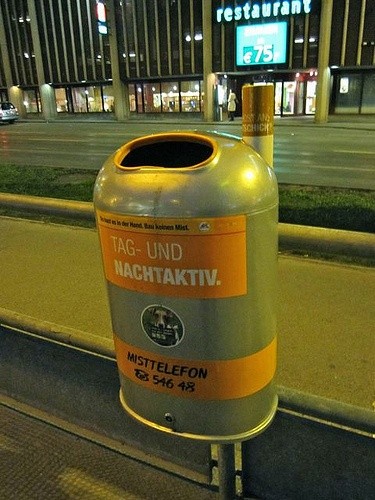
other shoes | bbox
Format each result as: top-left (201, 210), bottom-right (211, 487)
top-left (229, 119), bottom-right (234, 121)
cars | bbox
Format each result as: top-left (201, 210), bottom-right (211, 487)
top-left (0, 102), bottom-right (19, 125)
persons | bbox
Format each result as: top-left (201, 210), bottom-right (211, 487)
top-left (227, 88), bottom-right (236, 121)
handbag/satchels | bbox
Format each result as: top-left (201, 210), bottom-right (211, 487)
top-left (235, 99), bottom-right (240, 105)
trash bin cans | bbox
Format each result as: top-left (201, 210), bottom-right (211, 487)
top-left (92, 130), bottom-right (278, 445)
